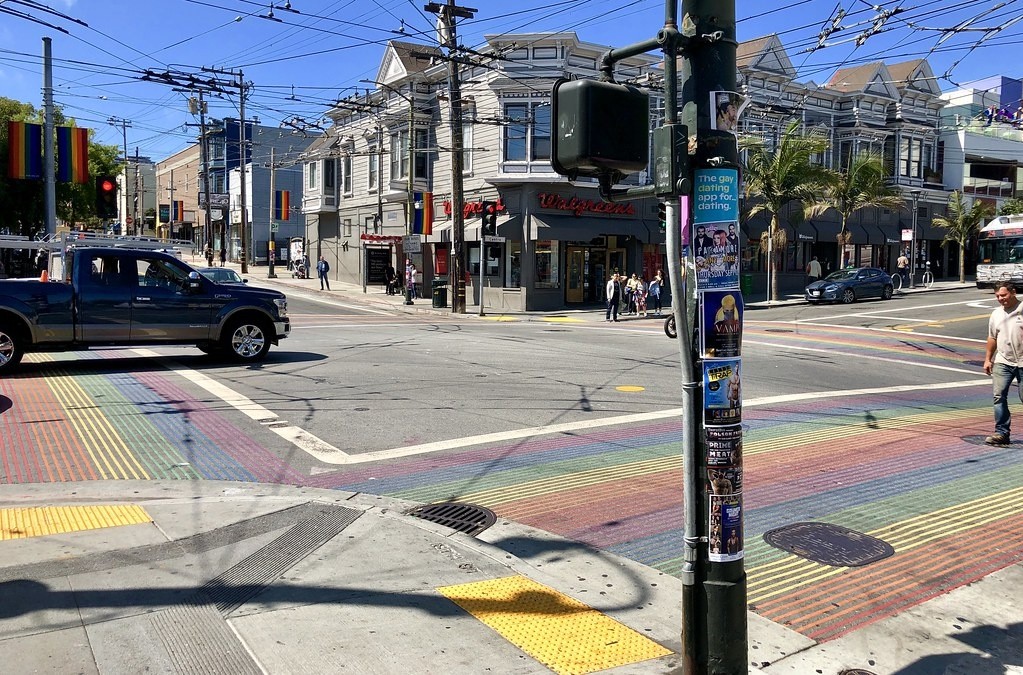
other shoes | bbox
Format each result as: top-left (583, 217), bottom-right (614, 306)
top-left (652, 311), bottom-right (665, 315)
top-left (320, 288), bottom-right (331, 291)
top-left (411, 296), bottom-right (417, 300)
top-left (386, 291), bottom-right (394, 296)
top-left (605, 314), bottom-right (620, 322)
top-left (220, 265), bottom-right (225, 267)
top-left (637, 313), bottom-right (647, 317)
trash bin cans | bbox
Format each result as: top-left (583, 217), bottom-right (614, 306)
top-left (431, 280), bottom-right (447, 306)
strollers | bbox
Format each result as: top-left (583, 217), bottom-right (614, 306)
top-left (292, 259), bottom-right (305, 279)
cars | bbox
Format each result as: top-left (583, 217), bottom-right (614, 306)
top-left (805, 267), bottom-right (894, 304)
top-left (115, 236), bottom-right (181, 256)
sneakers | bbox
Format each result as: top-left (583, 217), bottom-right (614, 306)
top-left (985, 433), bottom-right (1010, 444)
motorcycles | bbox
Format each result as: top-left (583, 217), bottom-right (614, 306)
top-left (664, 293), bottom-right (677, 339)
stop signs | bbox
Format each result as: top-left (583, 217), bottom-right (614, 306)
top-left (126, 218), bottom-right (133, 223)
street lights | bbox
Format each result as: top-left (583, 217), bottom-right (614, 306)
top-left (909, 190), bottom-right (921, 289)
top-left (358, 79), bottom-right (414, 305)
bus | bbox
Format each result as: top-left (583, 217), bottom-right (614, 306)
top-left (976, 214), bottom-right (1023, 294)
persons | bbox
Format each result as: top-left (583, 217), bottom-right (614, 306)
top-left (711, 496), bottom-right (721, 554)
top-left (807, 256), bottom-right (821, 285)
top-left (316, 256), bottom-right (330, 291)
top-left (409, 264), bottom-right (417, 299)
top-left (714, 295), bottom-right (741, 332)
top-left (844, 251), bottom-right (851, 269)
top-left (727, 529), bottom-right (738, 555)
top-left (715, 409), bottom-right (740, 418)
top-left (897, 253), bottom-right (909, 285)
top-left (984, 281), bottom-right (1023, 444)
top-left (385, 263), bottom-right (402, 296)
top-left (606, 269), bottom-right (666, 322)
top-left (204, 241), bottom-right (226, 267)
top-left (727, 363), bottom-right (740, 408)
top-left (731, 442), bottom-right (742, 464)
top-left (303, 252), bottom-right (310, 279)
top-left (718, 102), bottom-right (735, 131)
top-left (694, 224), bottom-right (738, 271)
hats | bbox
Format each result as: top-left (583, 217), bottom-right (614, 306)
top-left (638, 276), bottom-right (644, 280)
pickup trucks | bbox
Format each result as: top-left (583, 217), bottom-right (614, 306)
top-left (0, 231), bottom-right (292, 378)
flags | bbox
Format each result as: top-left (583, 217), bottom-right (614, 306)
top-left (56, 126), bottom-right (88, 183)
top-left (6, 121), bottom-right (42, 180)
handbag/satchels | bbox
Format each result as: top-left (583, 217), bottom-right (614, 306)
top-left (807, 262), bottom-right (811, 274)
top-left (626, 287), bottom-right (632, 292)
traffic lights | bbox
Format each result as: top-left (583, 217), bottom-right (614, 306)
top-left (96, 176), bottom-right (118, 219)
top-left (481, 201), bottom-right (497, 236)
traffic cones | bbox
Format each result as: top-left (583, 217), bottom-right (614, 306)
top-left (39, 270), bottom-right (48, 283)
